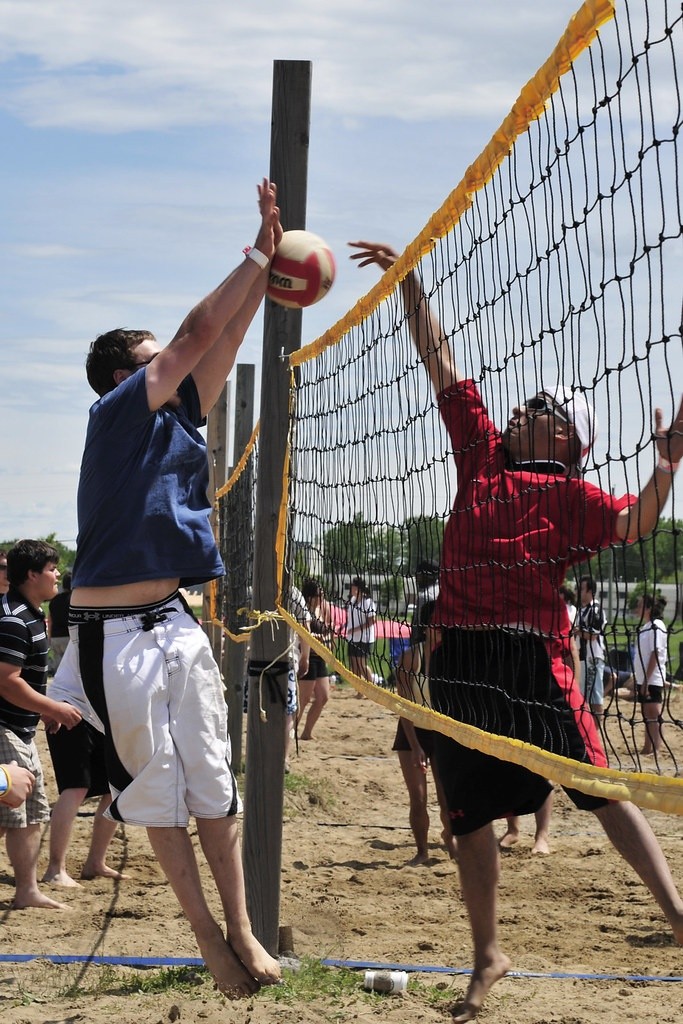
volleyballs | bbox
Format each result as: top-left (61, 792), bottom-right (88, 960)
top-left (266, 229), bottom-right (337, 307)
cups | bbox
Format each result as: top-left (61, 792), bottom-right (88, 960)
top-left (363, 970), bottom-right (408, 995)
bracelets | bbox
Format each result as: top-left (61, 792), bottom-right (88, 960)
top-left (656, 456), bottom-right (679, 474)
top-left (243, 246), bottom-right (269, 270)
top-left (0, 765), bottom-right (11, 798)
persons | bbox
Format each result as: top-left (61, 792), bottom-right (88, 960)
top-left (390, 600), bottom-right (457, 866)
top-left (408, 558), bottom-right (441, 644)
top-left (44, 639), bottom-right (131, 887)
top-left (48, 573), bottom-right (72, 661)
top-left (500, 778), bottom-right (554, 854)
top-left (291, 580), bottom-right (331, 739)
top-left (560, 576), bottom-right (607, 731)
top-left (348, 241), bottom-right (683, 1024)
top-left (346, 577), bottom-right (376, 699)
top-left (633, 594), bottom-right (667, 754)
top-left (0, 539), bottom-right (82, 910)
top-left (68, 177), bottom-right (282, 1000)
top-left (602, 667), bottom-right (683, 701)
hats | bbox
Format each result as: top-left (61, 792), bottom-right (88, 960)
top-left (539, 385), bottom-right (599, 480)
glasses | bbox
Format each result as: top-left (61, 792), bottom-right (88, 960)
top-left (522, 397), bottom-right (571, 426)
top-left (0, 565), bottom-right (7, 570)
top-left (124, 353), bottom-right (158, 368)
